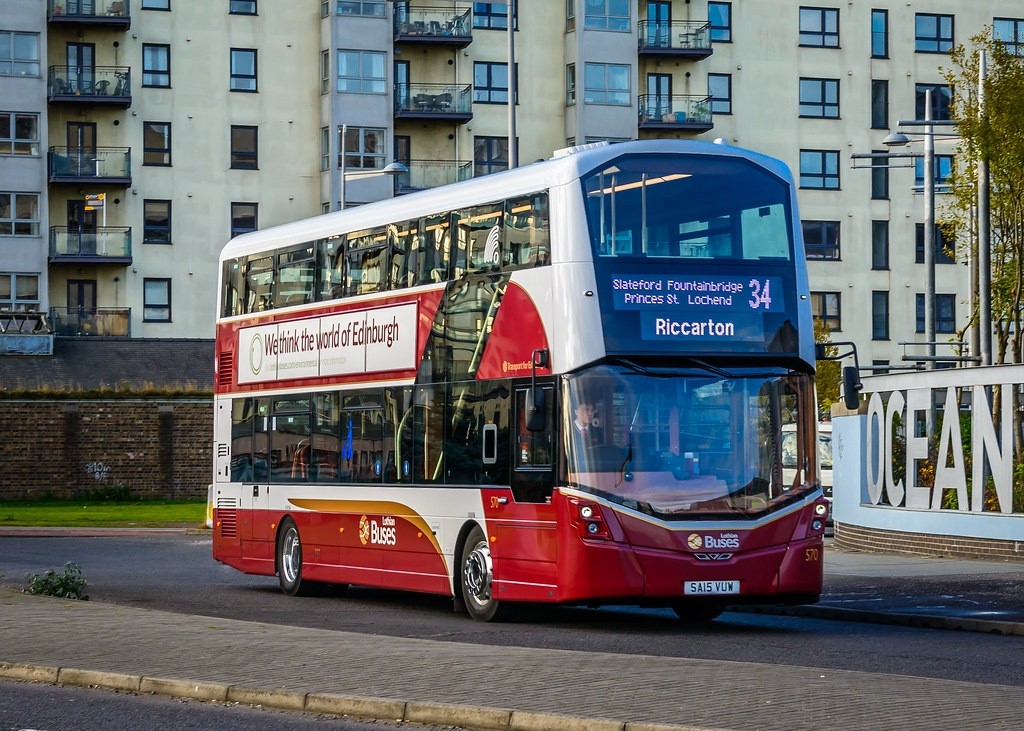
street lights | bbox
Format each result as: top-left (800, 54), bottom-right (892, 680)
top-left (882, 48), bottom-right (992, 512)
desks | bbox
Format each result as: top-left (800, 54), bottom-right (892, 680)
top-left (684, 33), bottom-right (698, 36)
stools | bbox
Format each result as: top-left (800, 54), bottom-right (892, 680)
top-left (679, 42), bottom-right (690, 47)
top-left (693, 38), bottom-right (702, 47)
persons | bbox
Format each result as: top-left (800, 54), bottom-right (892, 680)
top-left (572, 396), bottom-right (603, 447)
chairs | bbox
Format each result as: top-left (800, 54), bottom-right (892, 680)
top-left (58, 314), bottom-right (98, 335)
top-left (52, 78), bottom-right (73, 95)
top-left (412, 93), bottom-right (452, 112)
top-left (107, 1), bottom-right (123, 16)
top-left (414, 19), bottom-right (465, 35)
top-left (264, 267), bottom-right (445, 310)
top-left (83, 80), bottom-right (110, 95)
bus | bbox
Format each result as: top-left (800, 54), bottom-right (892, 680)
top-left (211, 136), bottom-right (863, 624)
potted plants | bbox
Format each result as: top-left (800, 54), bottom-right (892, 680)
top-left (690, 101), bottom-right (709, 122)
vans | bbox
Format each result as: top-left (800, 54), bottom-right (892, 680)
top-left (758, 420), bottom-right (832, 524)
